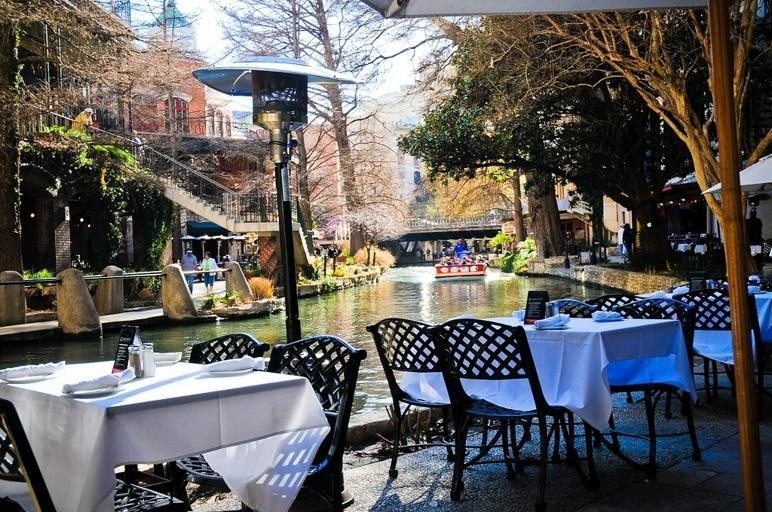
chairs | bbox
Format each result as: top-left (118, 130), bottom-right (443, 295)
top-left (668, 232), bottom-right (771, 276)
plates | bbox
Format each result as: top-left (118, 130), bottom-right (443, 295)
top-left (748, 290), bottom-right (766, 294)
top-left (69, 386), bottom-right (115, 398)
top-left (155, 360), bottom-right (179, 367)
top-left (592, 316), bottom-right (625, 322)
top-left (532, 326), bottom-right (569, 330)
top-left (209, 367), bottom-right (253, 377)
top-left (4, 373), bottom-right (50, 384)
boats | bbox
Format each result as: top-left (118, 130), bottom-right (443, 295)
top-left (433, 237), bottom-right (488, 280)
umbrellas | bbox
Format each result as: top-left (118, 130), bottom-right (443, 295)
top-left (700, 152), bottom-right (772, 195)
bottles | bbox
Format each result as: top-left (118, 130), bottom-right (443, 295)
top-left (545, 300), bottom-right (560, 319)
top-left (705, 279), bottom-right (711, 289)
top-left (128, 344), bottom-right (143, 378)
top-left (142, 343), bottom-right (156, 378)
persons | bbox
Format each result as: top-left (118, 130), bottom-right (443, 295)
top-left (197, 250), bottom-right (222, 294)
top-left (617, 223), bottom-right (626, 258)
top-left (179, 248), bottom-right (198, 295)
top-left (623, 224), bottom-right (632, 258)
